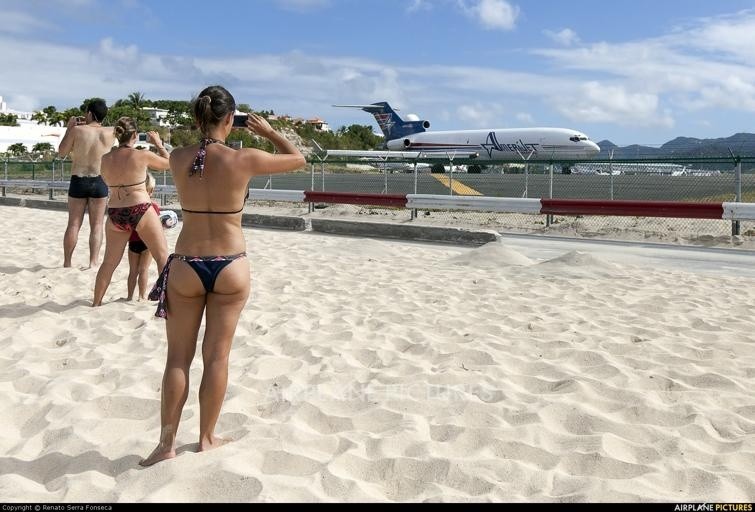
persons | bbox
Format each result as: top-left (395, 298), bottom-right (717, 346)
top-left (125, 172), bottom-right (161, 303)
top-left (57, 100), bottom-right (117, 269)
top-left (136, 84), bottom-right (309, 468)
top-left (89, 116), bottom-right (175, 306)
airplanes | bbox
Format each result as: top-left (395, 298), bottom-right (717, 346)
top-left (308, 102), bottom-right (602, 167)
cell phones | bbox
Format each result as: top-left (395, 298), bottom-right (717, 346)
top-left (230, 115), bottom-right (249, 128)
top-left (75, 117), bottom-right (80, 123)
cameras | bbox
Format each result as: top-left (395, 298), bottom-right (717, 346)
top-left (138, 133), bottom-right (149, 141)
